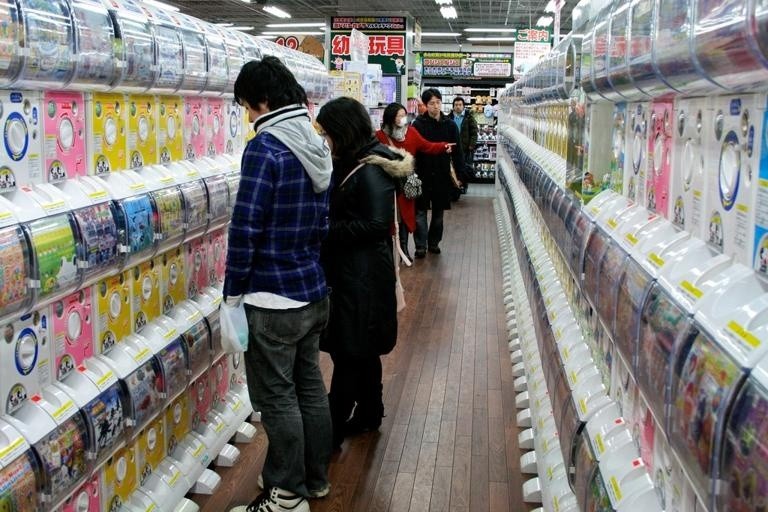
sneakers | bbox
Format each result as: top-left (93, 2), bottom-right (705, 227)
top-left (461, 187), bottom-right (467, 194)
top-left (414, 248), bottom-right (427, 259)
top-left (428, 244), bottom-right (441, 254)
top-left (344, 416), bottom-right (382, 434)
top-left (257, 473), bottom-right (333, 498)
top-left (403, 256), bottom-right (414, 265)
top-left (228, 486), bottom-right (311, 512)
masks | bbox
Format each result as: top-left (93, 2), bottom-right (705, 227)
top-left (396, 116), bottom-right (408, 127)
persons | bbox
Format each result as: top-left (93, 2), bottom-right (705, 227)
top-left (374, 100), bottom-right (457, 266)
top-left (221, 52), bottom-right (337, 512)
top-left (313, 94), bottom-right (416, 450)
top-left (410, 88), bottom-right (471, 258)
top-left (446, 96), bottom-right (479, 195)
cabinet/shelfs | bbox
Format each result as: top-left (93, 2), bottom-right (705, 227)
top-left (418, 78), bottom-right (512, 183)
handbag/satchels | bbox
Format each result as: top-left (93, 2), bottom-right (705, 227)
top-left (402, 173), bottom-right (423, 200)
